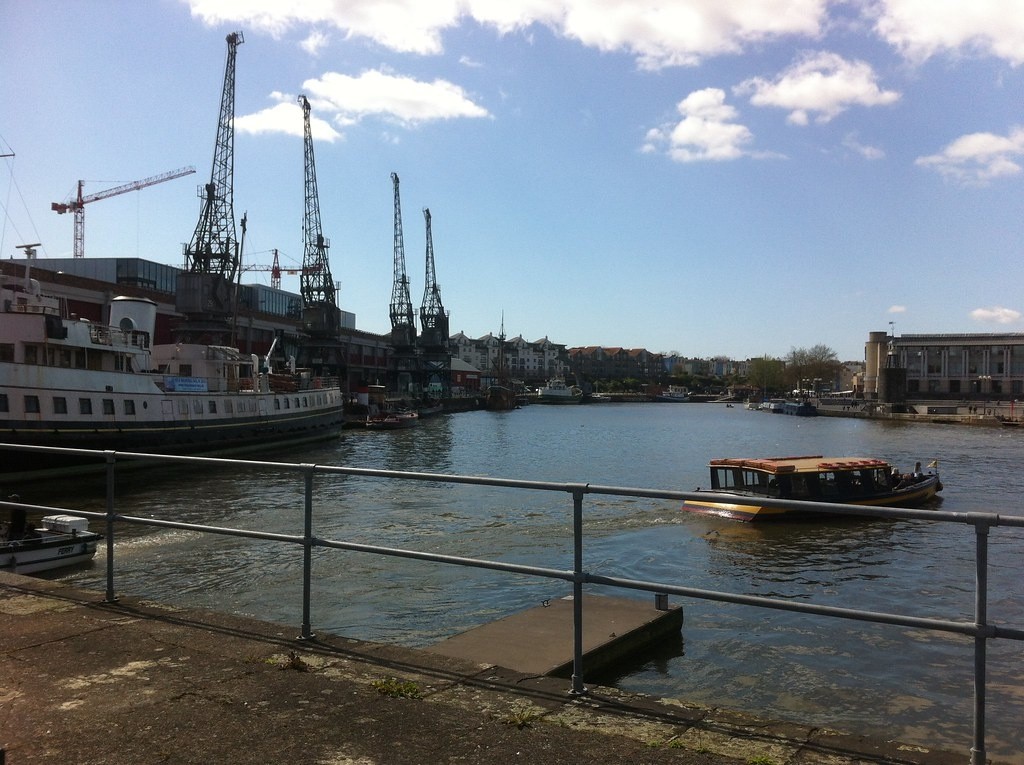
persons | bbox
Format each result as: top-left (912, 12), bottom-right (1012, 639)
top-left (969, 405), bottom-right (977, 414)
top-left (891, 468), bottom-right (911, 489)
top-left (769, 479), bottom-right (776, 488)
top-left (840, 471), bottom-right (861, 491)
top-left (914, 462), bottom-right (922, 482)
top-left (851, 400), bottom-right (858, 407)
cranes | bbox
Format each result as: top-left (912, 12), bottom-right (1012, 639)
top-left (421, 206), bottom-right (451, 349)
top-left (51, 165), bottom-right (196, 259)
top-left (176, 29), bottom-right (245, 318)
top-left (389, 170), bottom-right (417, 350)
top-left (297, 93), bottom-right (342, 337)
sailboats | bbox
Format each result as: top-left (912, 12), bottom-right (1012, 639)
top-left (485, 310), bottom-right (517, 411)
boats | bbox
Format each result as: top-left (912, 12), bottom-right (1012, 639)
top-left (0, 515), bottom-right (106, 575)
top-left (1, 130), bottom-right (345, 467)
top-left (537, 376), bottom-right (612, 403)
top-left (747, 390), bottom-right (818, 416)
top-left (366, 409), bottom-right (418, 429)
top-left (682, 454), bottom-right (945, 525)
top-left (662, 385), bottom-right (695, 398)
